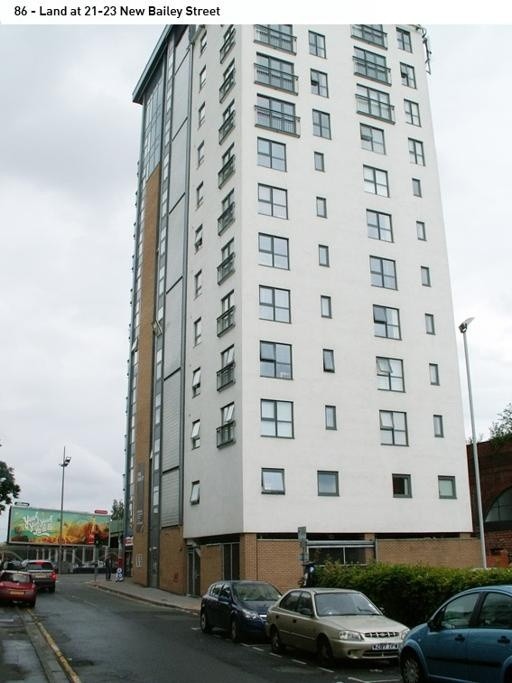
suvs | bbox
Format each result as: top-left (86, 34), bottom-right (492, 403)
top-left (22, 559), bottom-right (56, 593)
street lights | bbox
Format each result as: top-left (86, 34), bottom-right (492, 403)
top-left (58, 446), bottom-right (71, 574)
top-left (458, 316), bottom-right (487, 569)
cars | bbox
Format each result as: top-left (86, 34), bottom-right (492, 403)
top-left (0, 569), bottom-right (36, 608)
top-left (400, 585), bottom-right (512, 683)
top-left (201, 579), bottom-right (283, 641)
top-left (266, 588), bottom-right (410, 663)
